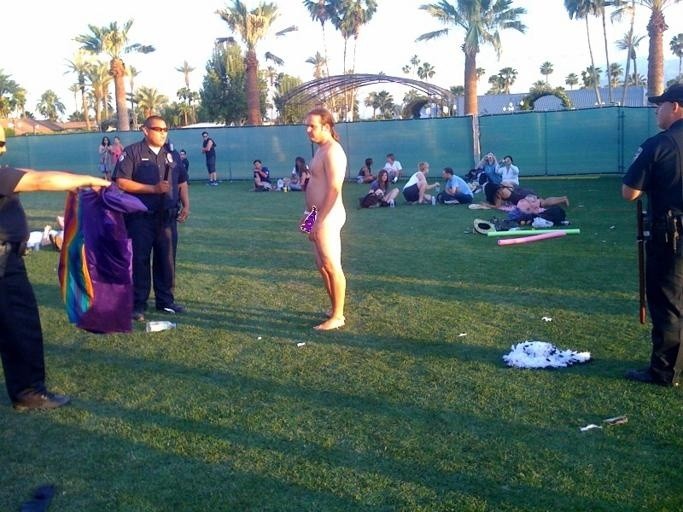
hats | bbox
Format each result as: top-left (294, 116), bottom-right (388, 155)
top-left (648, 84), bottom-right (683, 103)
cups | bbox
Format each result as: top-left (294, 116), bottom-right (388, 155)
top-left (33, 243), bottom-right (39, 252)
top-left (435, 185), bottom-right (440, 193)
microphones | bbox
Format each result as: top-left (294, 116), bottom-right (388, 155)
top-left (162, 164), bottom-right (170, 199)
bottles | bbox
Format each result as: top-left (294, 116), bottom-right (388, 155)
top-left (282, 187), bottom-right (287, 192)
top-left (431, 196), bottom-right (435, 205)
top-left (144, 320), bottom-right (175, 333)
top-left (389, 197), bottom-right (394, 207)
top-left (559, 220), bottom-right (572, 225)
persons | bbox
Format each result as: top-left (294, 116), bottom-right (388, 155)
top-left (114, 116), bottom-right (191, 325)
top-left (622, 81), bottom-right (683, 386)
top-left (305, 108), bottom-right (351, 331)
top-left (96, 128), bottom-right (569, 226)
top-left (0, 125), bottom-right (112, 412)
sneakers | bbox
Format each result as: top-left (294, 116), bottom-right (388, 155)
top-left (14, 391), bottom-right (70, 410)
top-left (133, 312), bottom-right (145, 321)
top-left (166, 302), bottom-right (185, 311)
top-left (626, 368), bottom-right (654, 382)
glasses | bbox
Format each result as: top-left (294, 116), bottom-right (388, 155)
top-left (150, 127), bottom-right (168, 132)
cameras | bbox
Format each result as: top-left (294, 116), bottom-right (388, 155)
top-left (501, 160), bottom-right (506, 163)
top-left (488, 156), bottom-right (493, 161)
